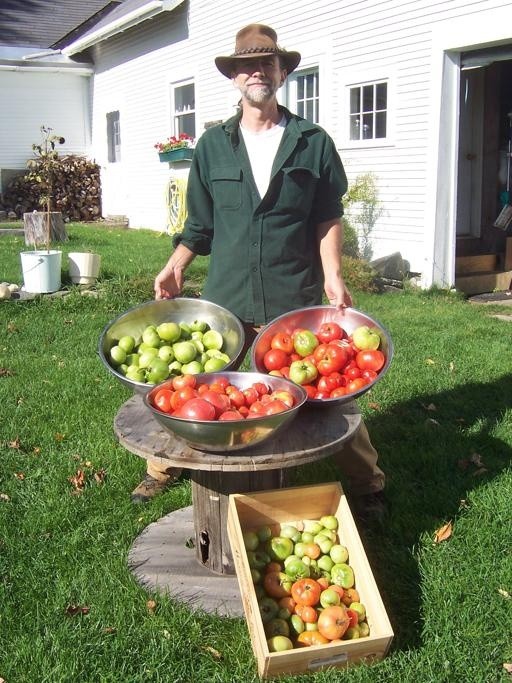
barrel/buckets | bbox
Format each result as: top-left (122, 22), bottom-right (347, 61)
top-left (19, 250), bottom-right (63, 294)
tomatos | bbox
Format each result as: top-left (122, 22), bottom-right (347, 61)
top-left (154, 375), bottom-right (293, 424)
top-left (263, 322), bottom-right (384, 399)
top-left (109, 319), bottom-right (231, 387)
top-left (243, 516), bottom-right (370, 653)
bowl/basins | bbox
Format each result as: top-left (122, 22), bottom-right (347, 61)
top-left (251, 303), bottom-right (393, 406)
top-left (97, 296), bottom-right (246, 395)
top-left (143, 370), bottom-right (308, 452)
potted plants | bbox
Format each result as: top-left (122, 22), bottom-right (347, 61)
top-left (19, 125), bottom-right (69, 294)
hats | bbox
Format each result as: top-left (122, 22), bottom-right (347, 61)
top-left (214, 24), bottom-right (302, 76)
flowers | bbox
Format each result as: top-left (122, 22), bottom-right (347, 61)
top-left (154, 133), bottom-right (193, 153)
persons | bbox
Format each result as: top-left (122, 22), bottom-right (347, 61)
top-left (125, 24), bottom-right (393, 523)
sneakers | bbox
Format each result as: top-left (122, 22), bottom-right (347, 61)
top-left (360, 492), bottom-right (384, 519)
top-left (130, 478), bottom-right (169, 501)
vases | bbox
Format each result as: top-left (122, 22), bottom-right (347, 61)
top-left (159, 147), bottom-right (194, 162)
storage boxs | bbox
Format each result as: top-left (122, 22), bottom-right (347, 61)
top-left (226, 481), bottom-right (394, 680)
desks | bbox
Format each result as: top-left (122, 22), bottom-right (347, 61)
top-left (112, 380), bottom-right (362, 620)
